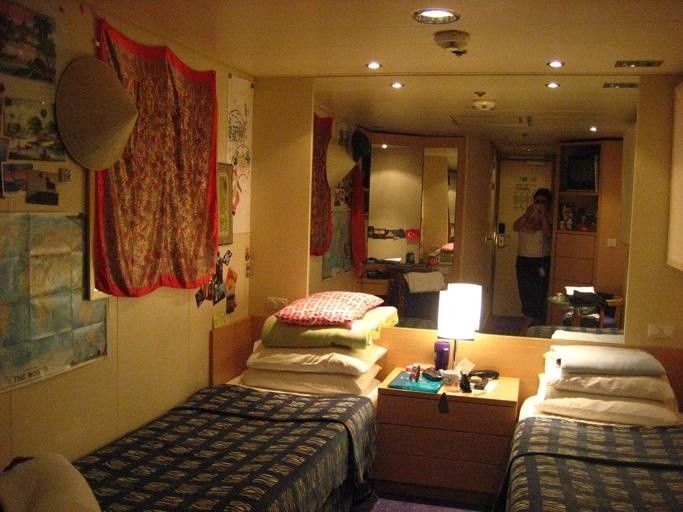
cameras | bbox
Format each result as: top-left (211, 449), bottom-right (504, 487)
top-left (532, 205), bottom-right (540, 210)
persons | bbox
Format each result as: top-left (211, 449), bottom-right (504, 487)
top-left (513, 188), bottom-right (552, 337)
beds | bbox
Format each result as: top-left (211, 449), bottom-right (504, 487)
top-left (63, 374), bottom-right (384, 511)
top-left (491, 392), bottom-right (682, 512)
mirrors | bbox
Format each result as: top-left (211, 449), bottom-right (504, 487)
top-left (362, 133), bottom-right (460, 266)
top-left (305, 75), bottom-right (642, 346)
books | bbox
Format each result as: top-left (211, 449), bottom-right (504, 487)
top-left (388, 370), bottom-right (444, 392)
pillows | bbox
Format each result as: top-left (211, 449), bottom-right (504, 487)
top-left (273, 289), bottom-right (385, 327)
top-left (541, 351), bottom-right (677, 400)
top-left (534, 372), bottom-right (682, 427)
top-left (548, 344), bottom-right (668, 376)
top-left (241, 364), bottom-right (383, 394)
top-left (244, 337), bottom-right (389, 375)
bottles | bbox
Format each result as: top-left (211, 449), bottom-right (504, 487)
top-left (560, 219), bottom-right (564, 230)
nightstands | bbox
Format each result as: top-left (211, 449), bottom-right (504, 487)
top-left (375, 366), bottom-right (520, 501)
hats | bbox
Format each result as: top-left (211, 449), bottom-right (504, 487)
top-left (55, 57), bottom-right (139, 172)
top-left (326, 138), bottom-right (356, 187)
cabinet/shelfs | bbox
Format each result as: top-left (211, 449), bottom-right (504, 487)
top-left (547, 138), bottom-right (623, 327)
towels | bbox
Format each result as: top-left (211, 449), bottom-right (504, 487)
top-left (400, 271), bottom-right (446, 293)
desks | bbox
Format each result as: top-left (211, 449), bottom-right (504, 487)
top-left (548, 292), bottom-right (624, 332)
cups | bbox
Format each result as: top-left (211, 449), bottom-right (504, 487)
top-left (405, 252), bottom-right (414, 264)
top-left (434, 341), bottom-right (450, 371)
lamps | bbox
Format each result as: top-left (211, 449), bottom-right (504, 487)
top-left (445, 282), bottom-right (483, 332)
top-left (437, 289), bottom-right (475, 372)
